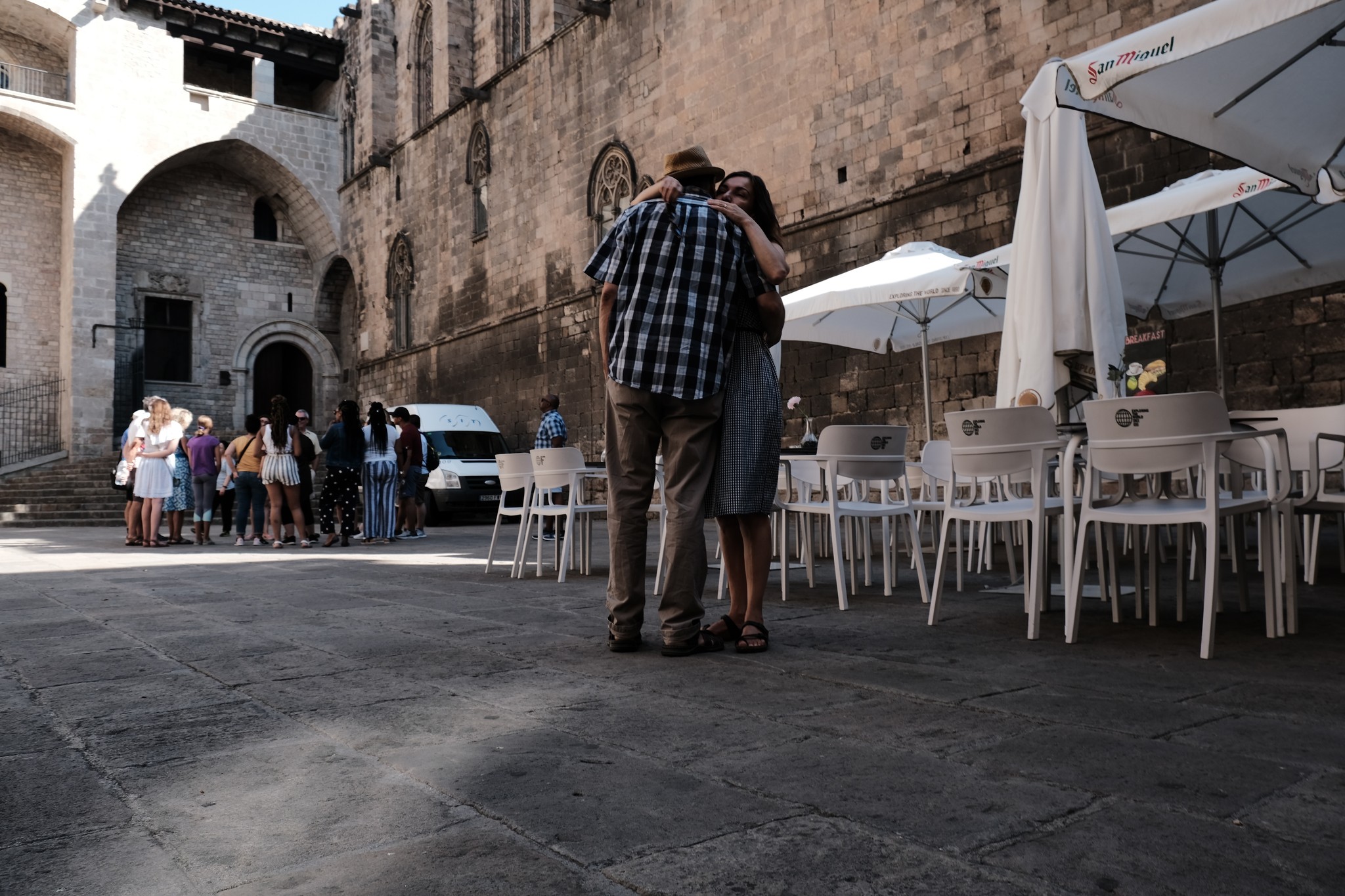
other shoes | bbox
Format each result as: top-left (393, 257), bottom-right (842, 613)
top-left (350, 526), bottom-right (429, 544)
top-left (308, 534), bottom-right (319, 543)
top-left (321, 533), bottom-right (339, 547)
top-left (531, 527), bottom-right (554, 540)
top-left (220, 531), bottom-right (230, 537)
top-left (194, 538), bottom-right (215, 545)
top-left (157, 533), bottom-right (169, 541)
top-left (543, 529), bottom-right (565, 540)
top-left (233, 533), bottom-right (297, 549)
top-left (340, 537), bottom-right (350, 547)
top-left (191, 528), bottom-right (204, 535)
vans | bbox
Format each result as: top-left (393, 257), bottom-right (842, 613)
top-left (366, 404), bottom-right (516, 528)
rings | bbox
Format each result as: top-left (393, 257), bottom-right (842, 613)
top-left (724, 204), bottom-right (727, 208)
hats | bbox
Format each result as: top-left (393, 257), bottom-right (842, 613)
top-left (132, 410), bottom-right (146, 420)
top-left (389, 407), bottom-right (409, 420)
top-left (656, 144), bottom-right (725, 183)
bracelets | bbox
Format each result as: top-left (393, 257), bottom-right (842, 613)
top-left (222, 486), bottom-right (227, 488)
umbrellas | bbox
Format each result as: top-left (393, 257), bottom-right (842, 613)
top-left (783, 241), bottom-right (1007, 549)
top-left (993, 55), bottom-right (1128, 424)
top-left (1056, 0), bottom-right (1345, 195)
top-left (960, 166), bottom-right (1345, 403)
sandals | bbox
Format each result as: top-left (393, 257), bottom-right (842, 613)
top-left (300, 540), bottom-right (313, 548)
top-left (661, 630), bottom-right (725, 656)
top-left (607, 629), bottom-right (641, 652)
top-left (734, 620), bottom-right (770, 653)
top-left (142, 539), bottom-right (170, 547)
top-left (700, 614), bottom-right (743, 638)
top-left (125, 536), bottom-right (143, 546)
top-left (166, 537), bottom-right (194, 544)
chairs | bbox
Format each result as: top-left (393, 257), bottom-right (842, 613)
top-left (485, 390), bottom-right (1345, 659)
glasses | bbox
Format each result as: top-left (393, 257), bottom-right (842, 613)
top-left (333, 410), bottom-right (339, 415)
top-left (541, 398), bottom-right (550, 403)
top-left (295, 415), bottom-right (306, 421)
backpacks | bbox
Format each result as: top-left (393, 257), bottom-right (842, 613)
top-left (420, 431), bottom-right (439, 472)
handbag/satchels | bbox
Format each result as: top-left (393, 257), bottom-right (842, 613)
top-left (110, 471), bottom-right (127, 491)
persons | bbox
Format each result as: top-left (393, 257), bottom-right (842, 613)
top-left (120, 394), bottom-right (430, 550)
top-left (581, 145), bottom-right (791, 654)
top-left (531, 394), bottom-right (567, 541)
